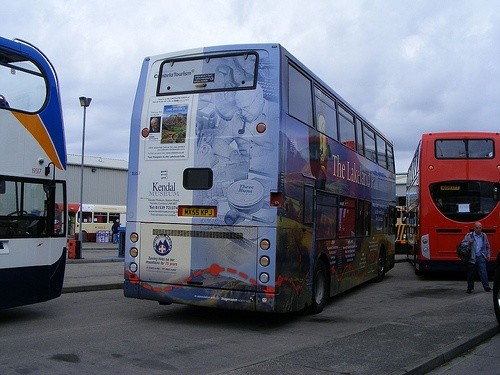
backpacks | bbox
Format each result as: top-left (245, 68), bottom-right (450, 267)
top-left (457, 231), bottom-right (474, 259)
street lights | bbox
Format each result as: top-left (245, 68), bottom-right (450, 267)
top-left (77, 95), bottom-right (93, 258)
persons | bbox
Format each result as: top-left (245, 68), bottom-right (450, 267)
top-left (112, 218), bottom-right (121, 244)
top-left (212, 81), bottom-right (278, 224)
top-left (461, 223), bottom-right (491, 293)
top-left (151, 118), bottom-right (160, 132)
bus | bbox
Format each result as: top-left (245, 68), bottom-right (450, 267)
top-left (73, 203), bottom-right (126, 243)
top-left (44, 200), bottom-right (80, 234)
top-left (0, 37), bottom-right (70, 311)
top-left (400, 132), bottom-right (500, 272)
top-left (122, 41), bottom-right (402, 316)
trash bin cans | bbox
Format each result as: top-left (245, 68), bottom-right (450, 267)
top-left (68, 240), bottom-right (77, 258)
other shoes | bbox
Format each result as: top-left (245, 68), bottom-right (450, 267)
top-left (466, 288), bottom-right (474, 293)
top-left (484, 287), bottom-right (492, 292)
